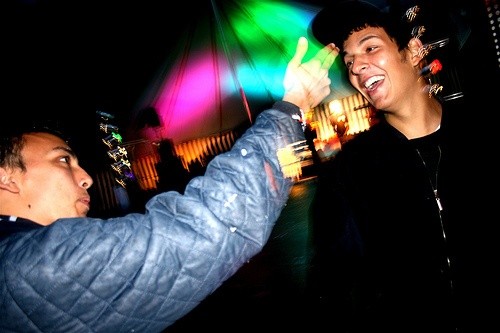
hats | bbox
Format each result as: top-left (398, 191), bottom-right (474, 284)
top-left (308, 0), bottom-right (413, 50)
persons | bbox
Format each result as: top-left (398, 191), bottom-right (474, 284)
top-left (0, 35), bottom-right (340, 332)
top-left (307, 5), bottom-right (500, 333)
